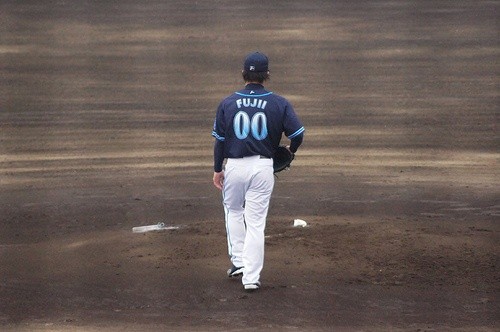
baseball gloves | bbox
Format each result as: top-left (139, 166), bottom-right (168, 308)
top-left (274, 144), bottom-right (295, 173)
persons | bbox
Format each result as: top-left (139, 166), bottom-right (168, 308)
top-left (212, 51), bottom-right (304, 290)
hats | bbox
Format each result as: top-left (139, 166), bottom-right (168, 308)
top-left (244, 53), bottom-right (268, 72)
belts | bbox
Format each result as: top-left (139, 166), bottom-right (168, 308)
top-left (231, 155), bottom-right (271, 160)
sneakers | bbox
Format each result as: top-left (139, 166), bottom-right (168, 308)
top-left (227, 265), bottom-right (244, 276)
top-left (244, 284), bottom-right (259, 290)
